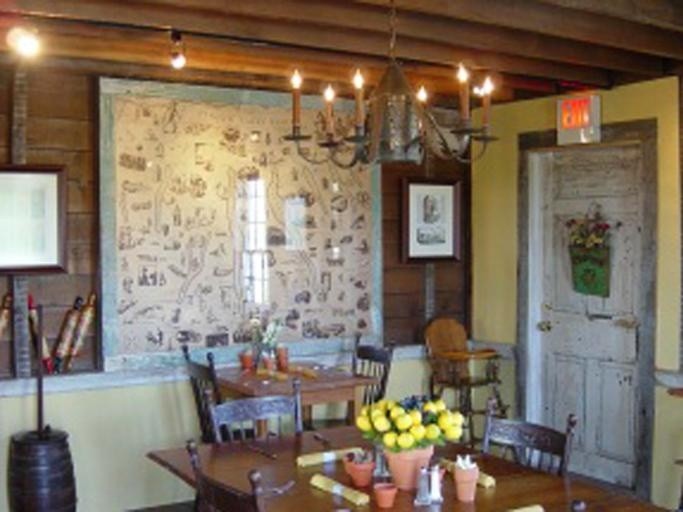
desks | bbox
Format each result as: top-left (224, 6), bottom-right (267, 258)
top-left (144, 425), bottom-right (667, 512)
top-left (215, 361), bottom-right (378, 440)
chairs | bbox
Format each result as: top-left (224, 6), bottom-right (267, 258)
top-left (183, 346), bottom-right (227, 512)
top-left (185, 440), bottom-right (267, 512)
top-left (424, 316), bottom-right (522, 463)
top-left (352, 333), bottom-right (396, 412)
top-left (205, 378), bottom-right (304, 442)
top-left (480, 398), bottom-right (578, 475)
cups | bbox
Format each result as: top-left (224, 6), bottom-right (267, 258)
top-left (453, 468), bottom-right (479, 502)
top-left (374, 483), bottom-right (398, 508)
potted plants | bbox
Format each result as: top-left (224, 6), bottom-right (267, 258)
top-left (347, 449), bottom-right (479, 509)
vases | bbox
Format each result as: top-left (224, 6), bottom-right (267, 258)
top-left (383, 446), bottom-right (433, 491)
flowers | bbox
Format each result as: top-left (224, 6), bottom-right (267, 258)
top-left (237, 320), bottom-right (290, 371)
top-left (558, 202), bottom-right (616, 248)
top-left (354, 396), bottom-right (466, 449)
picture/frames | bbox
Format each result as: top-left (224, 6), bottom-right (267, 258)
top-left (400, 177), bottom-right (463, 263)
top-left (0, 164), bottom-right (69, 275)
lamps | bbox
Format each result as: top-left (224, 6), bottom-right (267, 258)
top-left (169, 30), bottom-right (186, 71)
top-left (282, 1), bottom-right (499, 169)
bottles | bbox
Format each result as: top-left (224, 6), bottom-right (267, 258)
top-left (415, 466), bottom-right (431, 506)
top-left (430, 463), bottom-right (444, 503)
